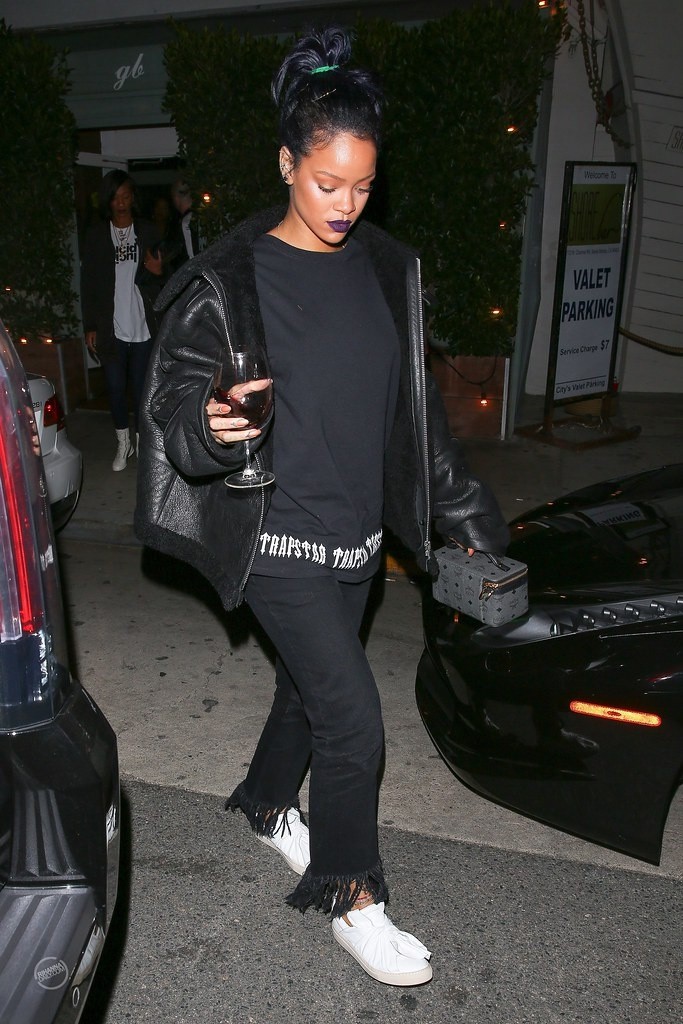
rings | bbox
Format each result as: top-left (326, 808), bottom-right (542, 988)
top-left (144, 262), bottom-right (146, 264)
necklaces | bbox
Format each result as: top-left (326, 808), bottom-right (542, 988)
top-left (112, 221), bottom-right (132, 259)
top-left (278, 220), bottom-right (346, 248)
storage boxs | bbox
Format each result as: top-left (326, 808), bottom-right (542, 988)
top-left (432, 545), bottom-right (529, 628)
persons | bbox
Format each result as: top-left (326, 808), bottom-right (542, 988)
top-left (82, 170), bottom-right (163, 471)
top-left (133, 29), bottom-right (510, 987)
top-left (153, 177), bottom-right (231, 285)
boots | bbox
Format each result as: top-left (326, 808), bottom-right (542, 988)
top-left (135, 433), bottom-right (139, 458)
top-left (112, 427), bottom-right (134, 472)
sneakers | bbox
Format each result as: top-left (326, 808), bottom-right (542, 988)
top-left (254, 807), bottom-right (311, 876)
top-left (331, 901), bottom-right (433, 986)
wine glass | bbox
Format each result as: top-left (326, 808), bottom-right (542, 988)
top-left (216, 345), bottom-right (274, 488)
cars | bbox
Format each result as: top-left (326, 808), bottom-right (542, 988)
top-left (22, 369), bottom-right (82, 535)
top-left (412, 461), bottom-right (683, 874)
top-left (0, 318), bottom-right (126, 1024)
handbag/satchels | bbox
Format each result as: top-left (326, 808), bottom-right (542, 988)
top-left (431, 542), bottom-right (529, 627)
